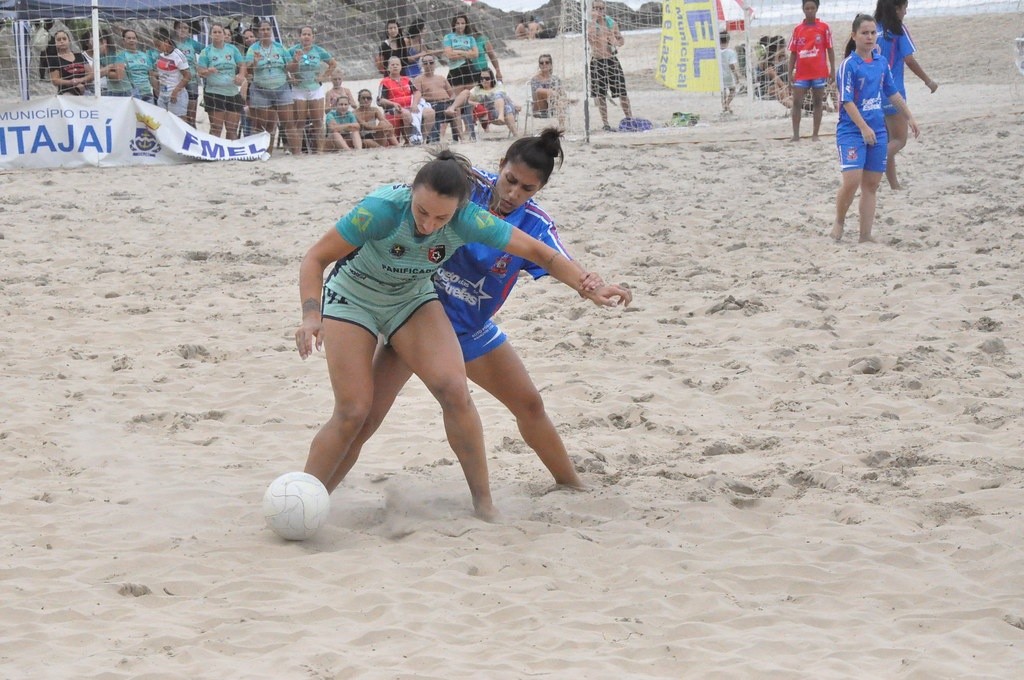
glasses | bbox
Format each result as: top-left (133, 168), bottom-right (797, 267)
top-left (423, 62), bottom-right (434, 65)
top-left (480, 77), bottom-right (491, 80)
top-left (593, 6), bottom-right (604, 10)
top-left (539, 61), bottom-right (550, 64)
top-left (360, 97), bottom-right (372, 100)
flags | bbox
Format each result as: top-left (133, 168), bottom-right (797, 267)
top-left (655, 0), bottom-right (724, 91)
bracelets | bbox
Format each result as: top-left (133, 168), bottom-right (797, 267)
top-left (925, 78), bottom-right (934, 86)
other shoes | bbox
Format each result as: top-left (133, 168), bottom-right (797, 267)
top-left (284, 149), bottom-right (291, 155)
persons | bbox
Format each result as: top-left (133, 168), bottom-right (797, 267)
top-left (788, 1), bottom-right (835, 144)
top-left (529, 54), bottom-right (583, 136)
top-left (833, 0), bottom-right (939, 245)
top-left (48, 12), bottom-right (521, 155)
top-left (515, 16), bottom-right (542, 39)
top-left (294, 127), bottom-right (632, 530)
top-left (584, 0), bottom-right (635, 132)
top-left (719, 0), bottom-right (836, 143)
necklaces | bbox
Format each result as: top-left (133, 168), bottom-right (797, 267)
top-left (260, 38), bottom-right (276, 72)
top-left (389, 72), bottom-right (405, 90)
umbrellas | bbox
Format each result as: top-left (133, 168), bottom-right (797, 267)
top-left (716, 0), bottom-right (754, 33)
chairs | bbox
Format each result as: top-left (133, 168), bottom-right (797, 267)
top-left (458, 80), bottom-right (570, 140)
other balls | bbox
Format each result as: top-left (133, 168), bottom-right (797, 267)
top-left (261, 471), bottom-right (332, 542)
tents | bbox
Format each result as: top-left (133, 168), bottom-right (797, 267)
top-left (0, 0), bottom-right (313, 155)
